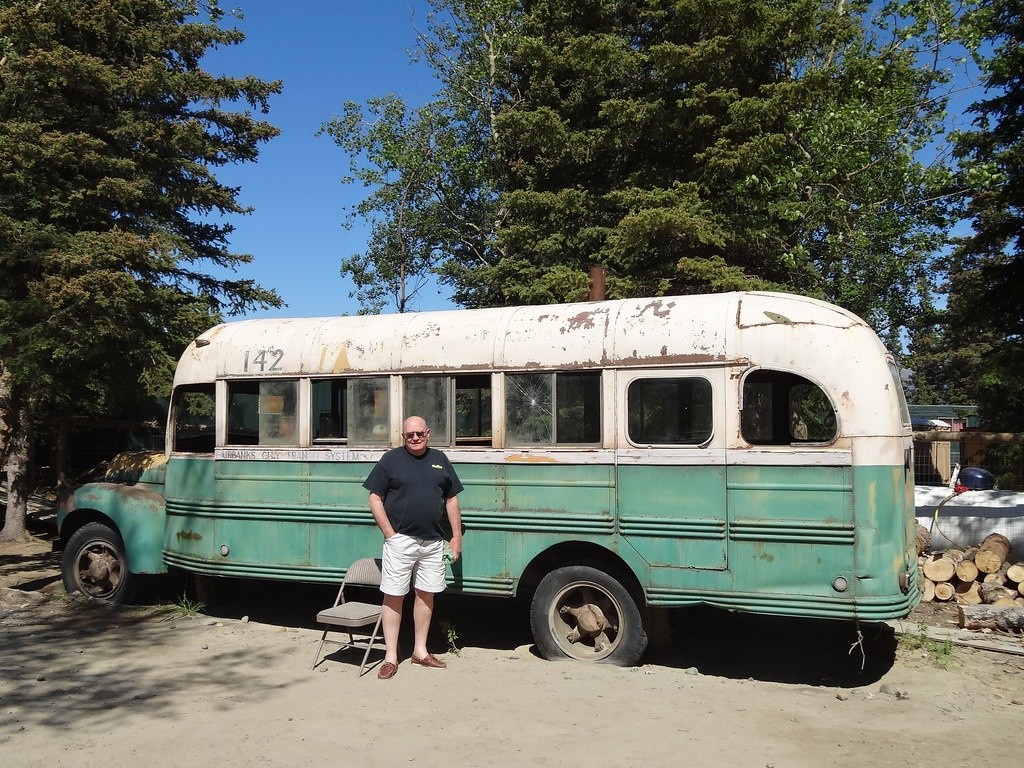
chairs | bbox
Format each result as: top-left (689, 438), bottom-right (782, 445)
top-left (311, 559), bottom-right (383, 680)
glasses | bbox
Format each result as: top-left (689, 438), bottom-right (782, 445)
top-left (403, 431), bottom-right (427, 438)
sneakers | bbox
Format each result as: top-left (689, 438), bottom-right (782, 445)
top-left (410, 653), bottom-right (447, 669)
top-left (378, 660), bottom-right (398, 679)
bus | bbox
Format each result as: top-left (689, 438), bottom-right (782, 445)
top-left (49, 292), bottom-right (924, 664)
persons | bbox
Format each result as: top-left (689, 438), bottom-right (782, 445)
top-left (361, 415), bottom-right (466, 681)
